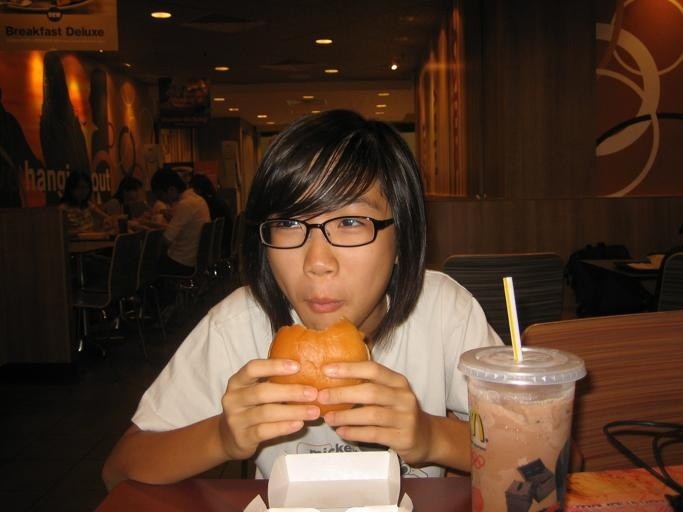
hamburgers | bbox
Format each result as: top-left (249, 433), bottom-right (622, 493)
top-left (268, 317), bottom-right (370, 418)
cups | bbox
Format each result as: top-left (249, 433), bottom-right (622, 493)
top-left (119, 214), bottom-right (128, 235)
top-left (457, 347), bottom-right (586, 512)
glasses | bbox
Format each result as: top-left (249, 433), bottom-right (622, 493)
top-left (259, 215), bottom-right (395, 249)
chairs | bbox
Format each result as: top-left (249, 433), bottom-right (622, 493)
top-left (74, 229), bottom-right (150, 361)
top-left (656, 254), bottom-right (683, 310)
top-left (522, 310), bottom-right (683, 472)
top-left (212, 216), bottom-right (227, 263)
top-left (118, 228), bottom-right (167, 359)
top-left (156, 222), bottom-right (214, 322)
top-left (442, 253), bottom-right (567, 345)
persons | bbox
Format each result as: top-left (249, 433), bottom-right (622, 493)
top-left (100, 107), bottom-right (506, 487)
top-left (148, 167), bottom-right (211, 270)
top-left (56, 170), bottom-right (110, 249)
top-left (103, 175), bottom-right (143, 225)
top-left (188, 175), bottom-right (234, 257)
